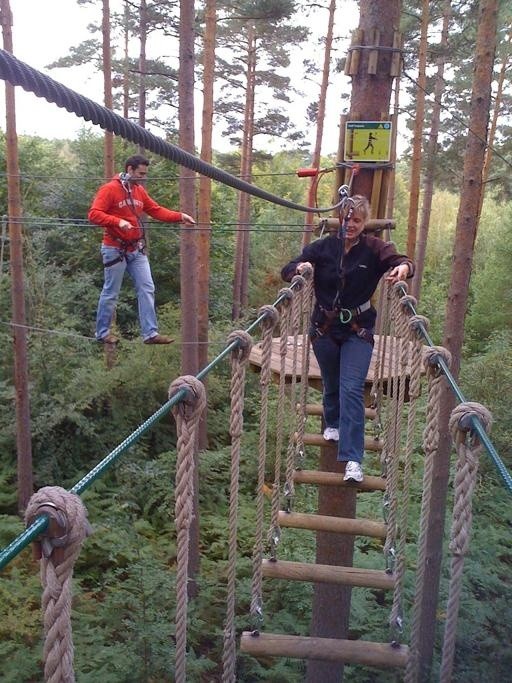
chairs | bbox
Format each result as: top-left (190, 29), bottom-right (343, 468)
top-left (144, 334), bottom-right (174, 344)
top-left (323, 426), bottom-right (339, 441)
top-left (101, 334), bottom-right (122, 344)
top-left (343, 461), bottom-right (364, 483)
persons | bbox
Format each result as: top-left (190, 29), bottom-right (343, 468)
top-left (280, 194), bottom-right (416, 483)
top-left (84, 154), bottom-right (197, 344)
